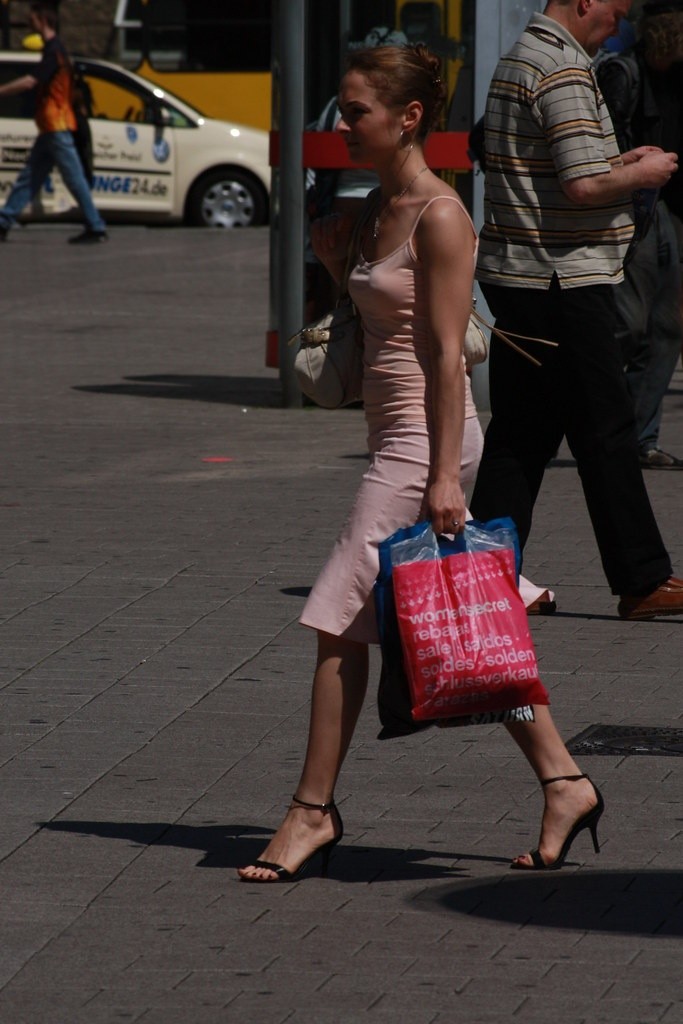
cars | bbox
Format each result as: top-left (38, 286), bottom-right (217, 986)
top-left (0, 51), bottom-right (270, 228)
top-left (107, 1), bottom-right (187, 70)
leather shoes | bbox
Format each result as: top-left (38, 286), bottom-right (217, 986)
top-left (517, 576), bottom-right (556, 614)
top-left (617, 576), bottom-right (683, 619)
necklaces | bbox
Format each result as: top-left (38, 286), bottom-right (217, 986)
top-left (371, 165), bottom-right (430, 240)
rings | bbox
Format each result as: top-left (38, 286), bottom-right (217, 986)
top-left (452, 520), bottom-right (461, 526)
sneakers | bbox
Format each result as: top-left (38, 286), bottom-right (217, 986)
top-left (638, 444), bottom-right (683, 469)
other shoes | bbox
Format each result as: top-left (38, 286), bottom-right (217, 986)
top-left (67, 230), bottom-right (108, 244)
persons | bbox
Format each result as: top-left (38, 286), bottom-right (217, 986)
top-left (307, 23), bottom-right (408, 216)
top-left (472, 0), bottom-right (683, 621)
top-left (0, 5), bottom-right (110, 243)
top-left (236, 43), bottom-right (606, 884)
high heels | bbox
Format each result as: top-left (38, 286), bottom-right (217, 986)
top-left (243, 794), bottom-right (343, 881)
top-left (510, 779), bottom-right (605, 869)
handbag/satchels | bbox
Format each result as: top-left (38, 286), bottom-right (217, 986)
top-left (287, 191), bottom-right (560, 409)
top-left (379, 517), bottom-right (550, 740)
